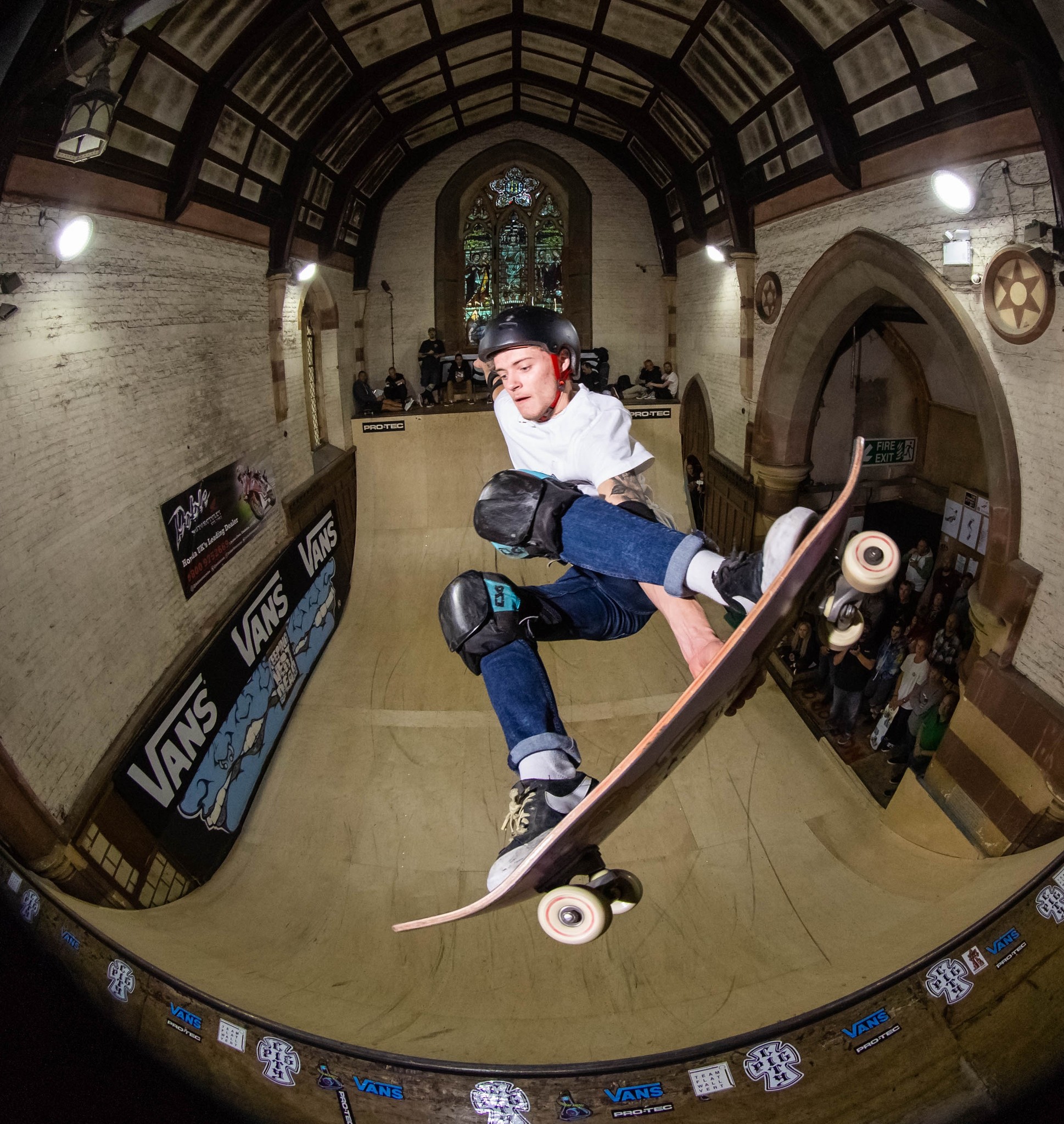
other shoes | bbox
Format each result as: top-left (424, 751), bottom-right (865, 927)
top-left (881, 746), bottom-right (907, 797)
top-left (864, 715), bottom-right (875, 723)
top-left (838, 734), bottom-right (850, 744)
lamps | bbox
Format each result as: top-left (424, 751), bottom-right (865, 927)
top-left (707, 240), bottom-right (734, 263)
top-left (0, 272), bottom-right (22, 320)
top-left (943, 229), bottom-right (973, 266)
top-left (54, 49), bottom-right (123, 164)
top-left (931, 158), bottom-right (1010, 214)
top-left (290, 260), bottom-right (318, 286)
top-left (1024, 219), bottom-right (1064, 272)
top-left (39, 212), bottom-right (95, 261)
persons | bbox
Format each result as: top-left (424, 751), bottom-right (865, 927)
top-left (383, 367), bottom-right (408, 404)
top-left (469, 301), bottom-right (820, 893)
top-left (774, 528), bottom-right (977, 797)
top-left (417, 327), bottom-right (446, 408)
top-left (444, 353), bottom-right (475, 406)
top-left (577, 358), bottom-right (679, 402)
top-left (686, 456), bottom-right (707, 530)
top-left (353, 371), bottom-right (416, 416)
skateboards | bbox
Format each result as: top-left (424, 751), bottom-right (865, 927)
top-left (438, 384), bottom-right (444, 406)
top-left (392, 435), bottom-right (898, 946)
top-left (866, 695), bottom-right (901, 751)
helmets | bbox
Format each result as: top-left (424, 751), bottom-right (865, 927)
top-left (479, 306), bottom-right (581, 380)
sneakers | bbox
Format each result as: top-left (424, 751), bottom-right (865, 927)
top-left (404, 393), bottom-right (493, 411)
top-left (711, 506), bottom-right (820, 616)
top-left (611, 387), bottom-right (656, 400)
top-left (486, 772), bottom-right (601, 892)
top-left (789, 653), bottom-right (794, 662)
